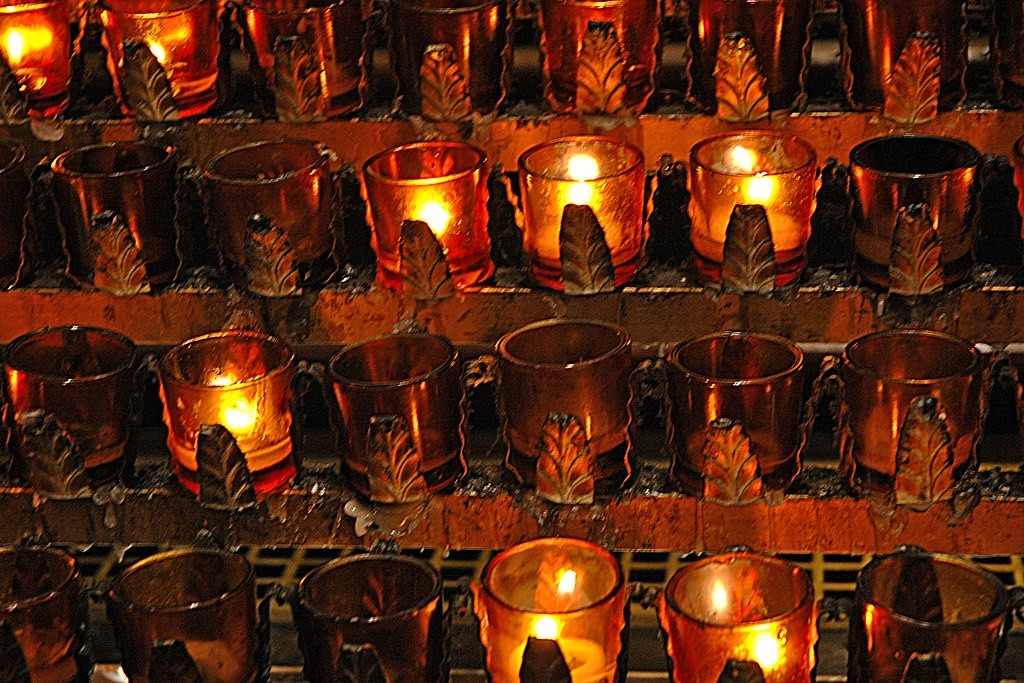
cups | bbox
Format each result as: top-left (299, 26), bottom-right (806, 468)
top-left (0, 546), bottom-right (1012, 683)
top-left (0, 132), bottom-right (1024, 298)
top-left (0, 0), bottom-right (1024, 123)
top-left (0, 322), bottom-right (992, 513)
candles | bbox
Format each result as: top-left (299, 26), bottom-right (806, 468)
top-left (383, 217), bottom-right (810, 286)
top-left (172, 436), bottom-right (292, 483)
top-left (512, 635), bottom-right (608, 683)
top-left (3, 32), bottom-right (210, 122)
